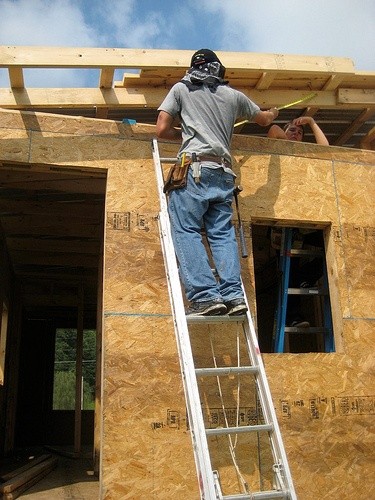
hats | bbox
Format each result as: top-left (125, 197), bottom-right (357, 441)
top-left (191, 48), bottom-right (227, 71)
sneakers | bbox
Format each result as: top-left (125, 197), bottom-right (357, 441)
top-left (185, 300), bottom-right (227, 316)
top-left (222, 298), bottom-right (248, 315)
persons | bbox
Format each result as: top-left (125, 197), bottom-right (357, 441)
top-left (155, 47), bottom-right (281, 318)
top-left (267, 116), bottom-right (331, 328)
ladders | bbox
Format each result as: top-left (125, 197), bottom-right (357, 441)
top-left (151, 137), bottom-right (299, 500)
top-left (274, 227), bottom-right (336, 352)
